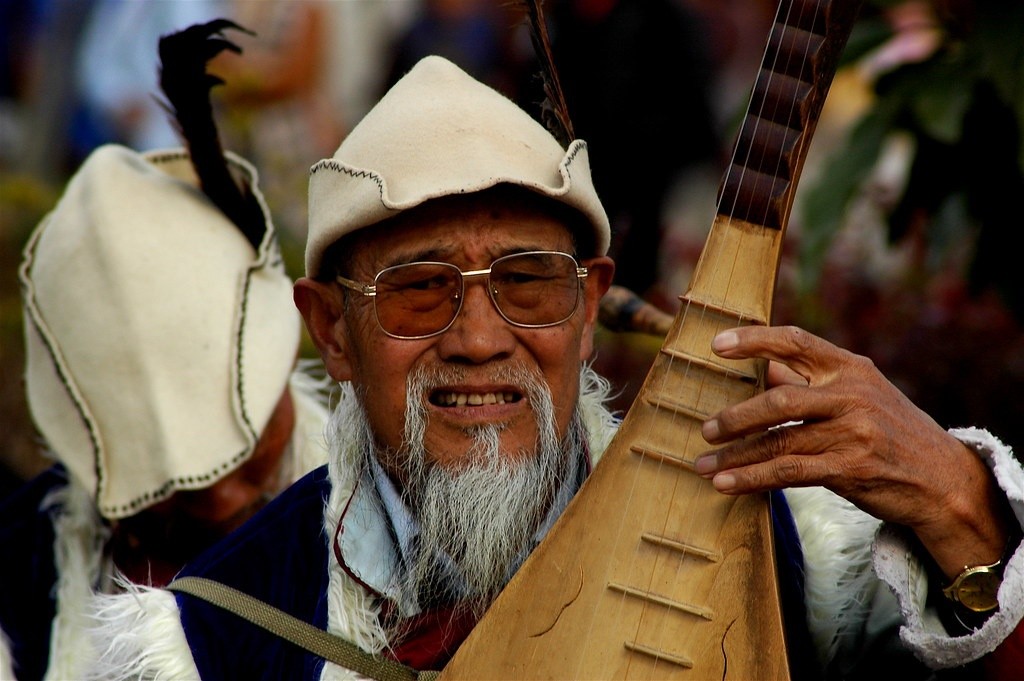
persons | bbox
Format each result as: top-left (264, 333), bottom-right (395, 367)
top-left (0, 139), bottom-right (333, 680)
top-left (86, 53), bottom-right (1024, 681)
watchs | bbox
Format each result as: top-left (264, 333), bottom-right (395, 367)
top-left (941, 549), bottom-right (1008, 627)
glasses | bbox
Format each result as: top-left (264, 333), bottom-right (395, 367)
top-left (335, 251), bottom-right (588, 341)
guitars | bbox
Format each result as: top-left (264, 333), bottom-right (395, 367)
top-left (430, 1), bottom-right (871, 680)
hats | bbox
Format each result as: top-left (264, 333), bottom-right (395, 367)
top-left (305, 54), bottom-right (611, 283)
top-left (17, 141), bottom-right (298, 519)
